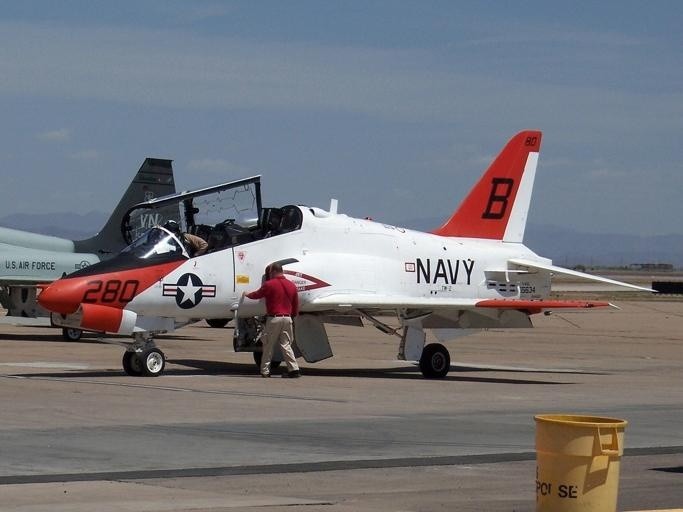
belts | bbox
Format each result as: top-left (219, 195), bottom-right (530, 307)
top-left (267, 314), bottom-right (291, 317)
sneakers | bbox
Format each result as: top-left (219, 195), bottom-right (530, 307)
top-left (261, 372), bottom-right (271, 378)
top-left (281, 370), bottom-right (301, 379)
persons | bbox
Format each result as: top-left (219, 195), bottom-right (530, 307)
top-left (155, 219), bottom-right (209, 258)
top-left (244, 263), bottom-right (303, 378)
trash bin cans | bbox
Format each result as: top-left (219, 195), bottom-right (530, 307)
top-left (533, 414), bottom-right (628, 512)
